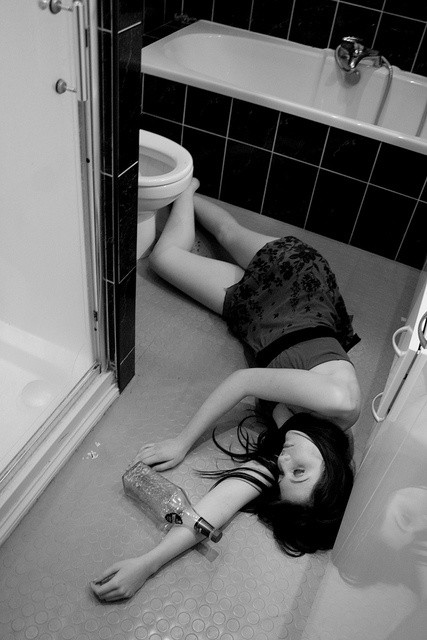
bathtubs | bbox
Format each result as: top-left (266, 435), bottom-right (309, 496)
top-left (139, 20), bottom-right (426, 154)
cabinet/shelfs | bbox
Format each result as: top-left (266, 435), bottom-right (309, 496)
top-left (359, 278), bottom-right (427, 453)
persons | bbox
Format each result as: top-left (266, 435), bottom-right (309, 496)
top-left (89, 176), bottom-right (362, 603)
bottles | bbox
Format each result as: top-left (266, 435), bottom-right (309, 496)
top-left (122, 461), bottom-right (223, 543)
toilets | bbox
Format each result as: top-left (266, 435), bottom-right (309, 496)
top-left (137, 128), bottom-right (194, 261)
